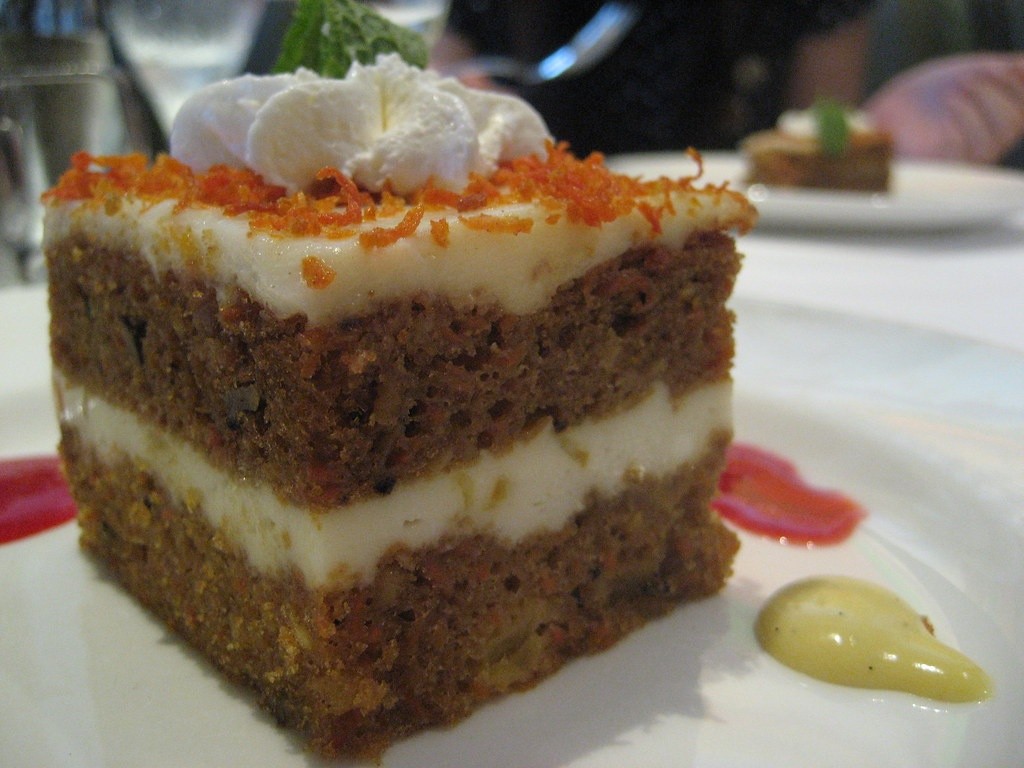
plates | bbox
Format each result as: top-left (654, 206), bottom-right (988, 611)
top-left (1, 296), bottom-right (1024, 768)
top-left (605, 156), bottom-right (1023, 230)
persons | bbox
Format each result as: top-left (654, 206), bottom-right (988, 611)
top-left (427, 0), bottom-right (1024, 166)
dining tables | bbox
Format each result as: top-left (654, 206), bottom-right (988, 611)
top-left (0, 153), bottom-right (1024, 768)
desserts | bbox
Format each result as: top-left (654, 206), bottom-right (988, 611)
top-left (41, 48), bottom-right (754, 752)
top-left (742, 100), bottom-right (893, 197)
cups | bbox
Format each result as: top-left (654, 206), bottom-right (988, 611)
top-left (103, 0), bottom-right (265, 159)
top-left (2, 33), bottom-right (126, 288)
